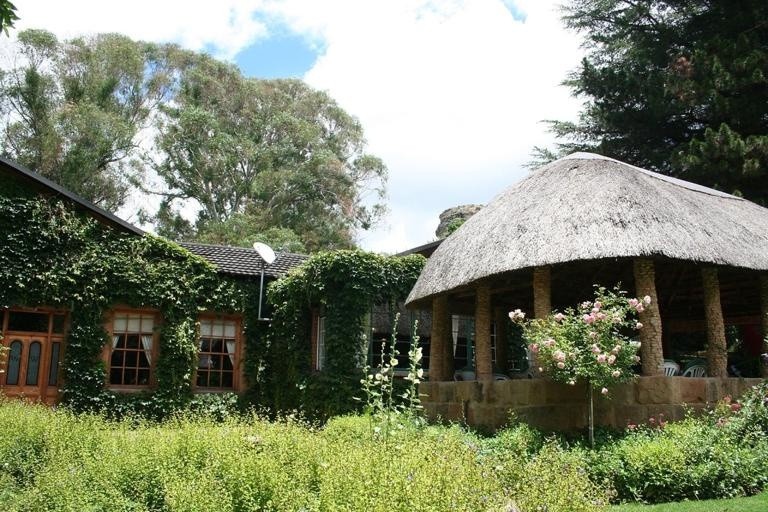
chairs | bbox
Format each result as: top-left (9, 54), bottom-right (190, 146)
top-left (453, 370), bottom-right (474, 381)
top-left (665, 361), bottom-right (680, 377)
top-left (681, 364), bottom-right (709, 377)
top-left (493, 373), bottom-right (509, 382)
